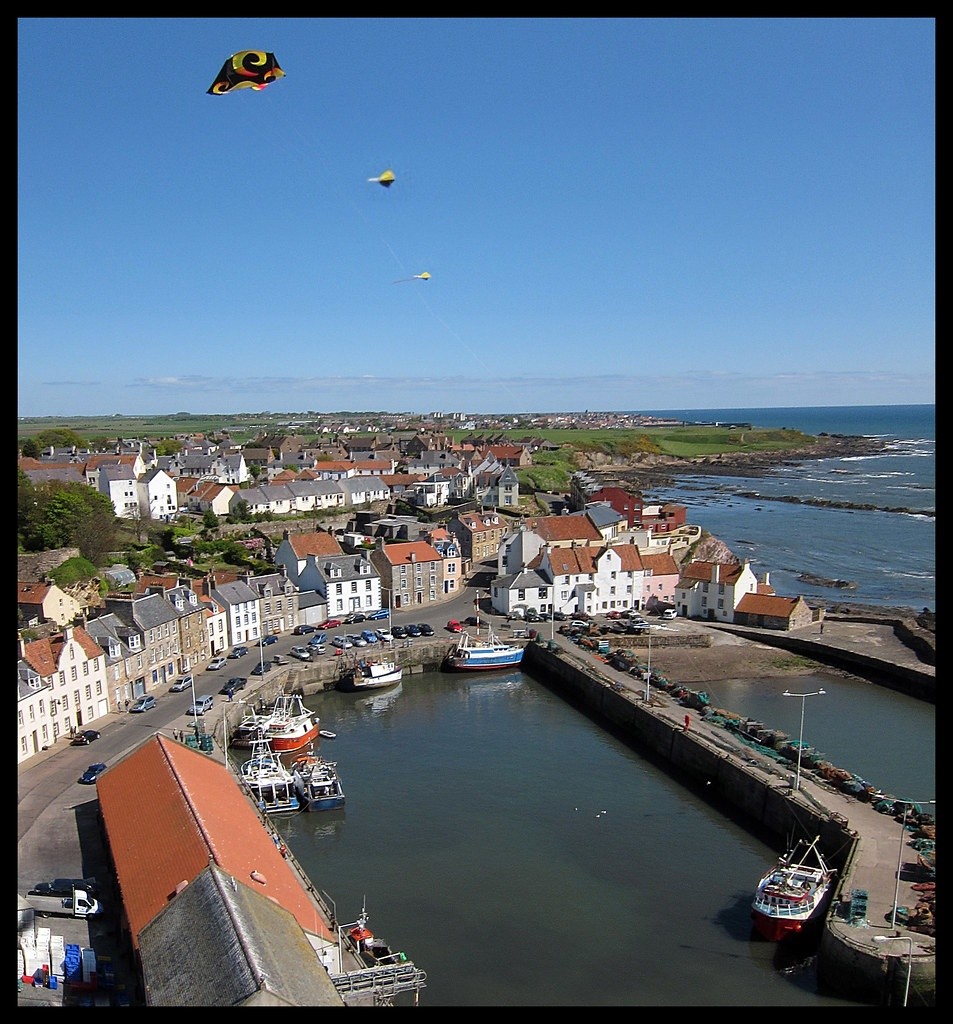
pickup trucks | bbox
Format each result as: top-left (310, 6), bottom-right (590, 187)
top-left (222, 677), bottom-right (247, 695)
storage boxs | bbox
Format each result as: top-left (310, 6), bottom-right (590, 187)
top-left (18, 926), bottom-right (131, 1007)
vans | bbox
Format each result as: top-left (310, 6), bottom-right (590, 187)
top-left (663, 609), bottom-right (678, 620)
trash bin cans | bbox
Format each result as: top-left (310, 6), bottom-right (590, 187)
top-left (790, 775), bottom-right (797, 790)
top-left (549, 639), bottom-right (554, 652)
top-left (537, 634), bottom-right (544, 644)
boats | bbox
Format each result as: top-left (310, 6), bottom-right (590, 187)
top-left (240, 727), bottom-right (301, 818)
top-left (320, 730), bottom-right (337, 738)
top-left (441, 624), bottom-right (525, 673)
top-left (334, 652), bottom-right (403, 692)
top-left (264, 694), bottom-right (320, 752)
top-left (291, 738), bottom-right (346, 812)
top-left (750, 835), bottom-right (839, 943)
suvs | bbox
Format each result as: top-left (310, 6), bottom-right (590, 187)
top-left (571, 621), bottom-right (589, 627)
top-left (189, 694), bottom-right (214, 715)
top-left (129, 694), bottom-right (157, 712)
top-left (170, 675), bottom-right (192, 693)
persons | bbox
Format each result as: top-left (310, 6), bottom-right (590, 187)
top-left (367, 660), bottom-right (370, 667)
top-left (70, 726), bottom-right (76, 737)
top-left (173, 728), bottom-right (178, 740)
top-left (821, 623), bottom-right (824, 633)
top-left (630, 537), bottom-right (634, 544)
top-left (801, 877), bottom-right (811, 889)
top-left (228, 689), bottom-right (233, 702)
top-left (125, 699), bottom-right (129, 713)
top-left (180, 730), bottom-right (183, 742)
top-left (359, 659), bottom-right (363, 668)
top-left (118, 701), bottom-right (121, 713)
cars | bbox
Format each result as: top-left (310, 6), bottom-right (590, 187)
top-left (73, 730), bottom-right (101, 745)
top-left (506, 612), bottom-right (567, 622)
top-left (361, 630), bottom-right (378, 643)
top-left (375, 629), bottom-right (394, 642)
top-left (607, 610), bottom-right (634, 619)
top-left (368, 609), bottom-right (392, 620)
top-left (80, 762), bottom-right (107, 784)
top-left (346, 634), bottom-right (367, 647)
top-left (290, 646), bottom-right (310, 659)
top-left (251, 661), bottom-right (272, 675)
top-left (345, 614), bottom-right (366, 624)
top-left (447, 619), bottom-right (463, 633)
top-left (417, 623), bottom-right (435, 636)
top-left (332, 635), bottom-right (353, 649)
top-left (404, 624), bottom-right (422, 637)
top-left (318, 619), bottom-right (341, 630)
top-left (257, 635), bottom-right (278, 646)
top-left (228, 647), bottom-right (248, 659)
top-left (294, 625), bottom-right (317, 635)
top-left (460, 616), bottom-right (487, 626)
top-left (391, 626), bottom-right (409, 639)
top-left (306, 632), bottom-right (328, 655)
top-left (572, 614), bottom-right (589, 621)
top-left (207, 657), bottom-right (227, 670)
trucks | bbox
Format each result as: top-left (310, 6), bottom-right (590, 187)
top-left (25, 887), bottom-right (105, 921)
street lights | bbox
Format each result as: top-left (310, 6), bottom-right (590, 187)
top-left (379, 587), bottom-right (399, 651)
top-left (782, 690), bottom-right (827, 798)
top-left (875, 795), bottom-right (936, 929)
top-left (635, 623), bottom-right (668, 701)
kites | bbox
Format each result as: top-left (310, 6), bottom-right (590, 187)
top-left (367, 170), bottom-right (396, 188)
top-left (393, 272), bottom-right (430, 286)
top-left (205, 49), bottom-right (286, 96)
